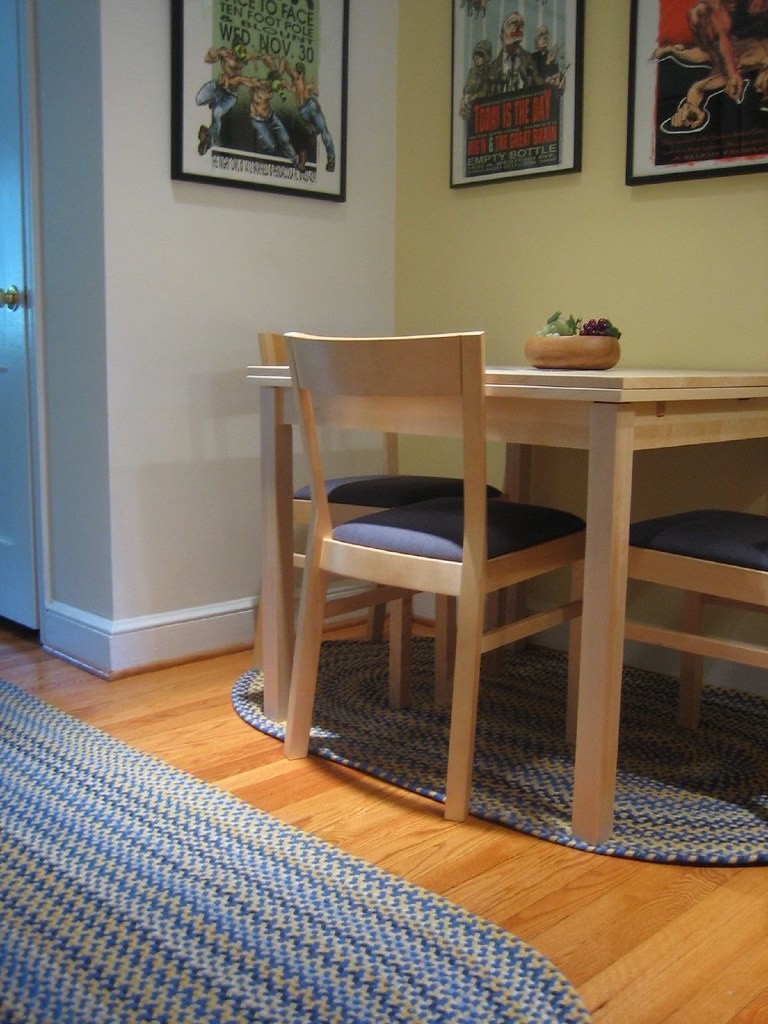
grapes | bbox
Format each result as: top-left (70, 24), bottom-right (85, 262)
top-left (536, 318), bottom-right (609, 336)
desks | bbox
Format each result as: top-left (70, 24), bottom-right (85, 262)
top-left (241, 366), bottom-right (768, 846)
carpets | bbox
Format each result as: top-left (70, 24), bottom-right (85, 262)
top-left (2, 674), bottom-right (587, 1022)
top-left (231, 631), bottom-right (768, 866)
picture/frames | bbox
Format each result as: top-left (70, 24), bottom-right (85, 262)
top-left (168, 0), bottom-right (350, 204)
top-left (623, 1), bottom-right (768, 187)
top-left (447, 1), bottom-right (585, 190)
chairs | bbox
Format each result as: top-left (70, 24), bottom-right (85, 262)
top-left (278, 329), bottom-right (590, 824)
top-left (249, 333), bottom-right (424, 708)
top-left (625, 506), bottom-right (767, 729)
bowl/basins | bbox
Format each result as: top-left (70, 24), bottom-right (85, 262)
top-left (525, 337), bottom-right (621, 370)
